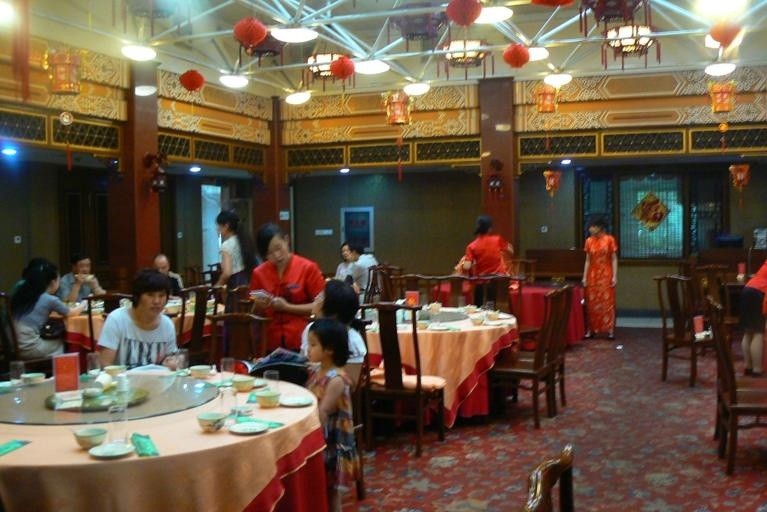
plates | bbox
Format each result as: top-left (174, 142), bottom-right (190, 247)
top-left (87, 444), bottom-right (135, 459)
top-left (278, 395), bottom-right (311, 408)
top-left (227, 422), bottom-right (269, 435)
top-left (497, 313), bottom-right (513, 319)
top-left (254, 378), bottom-right (267, 389)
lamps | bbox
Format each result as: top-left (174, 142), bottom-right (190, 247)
top-left (472, 6), bottom-right (515, 28)
top-left (133, 82), bottom-right (159, 99)
top-left (350, 53), bottom-right (392, 78)
top-left (284, 83), bottom-right (313, 105)
top-left (702, 30), bottom-right (745, 52)
top-left (483, 154), bottom-right (509, 196)
top-left (702, 60), bottom-right (738, 78)
top-left (121, 37), bottom-right (160, 63)
top-left (401, 81), bottom-right (433, 99)
top-left (543, 72), bottom-right (574, 93)
top-left (218, 66), bottom-right (251, 90)
top-left (303, 50), bottom-right (357, 92)
top-left (524, 45), bottom-right (550, 65)
top-left (437, 38), bottom-right (495, 80)
top-left (599, 24), bottom-right (663, 69)
top-left (270, 25), bottom-right (320, 47)
top-left (140, 149), bottom-right (172, 192)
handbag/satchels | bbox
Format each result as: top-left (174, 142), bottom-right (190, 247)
top-left (40, 317), bottom-right (66, 339)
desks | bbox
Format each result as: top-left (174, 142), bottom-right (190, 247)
top-left (301, 306), bottom-right (517, 428)
top-left (1, 366), bottom-right (326, 512)
top-left (40, 294), bottom-right (225, 365)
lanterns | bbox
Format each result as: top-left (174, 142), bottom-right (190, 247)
top-left (706, 79), bottom-right (735, 150)
top-left (234, 16), bottom-right (267, 56)
top-left (444, 0), bottom-right (482, 37)
top-left (709, 20), bottom-right (740, 47)
top-left (330, 55), bottom-right (353, 83)
top-left (543, 171), bottom-right (562, 197)
top-left (532, 84), bottom-right (559, 150)
top-left (729, 164), bottom-right (751, 210)
top-left (503, 41), bottom-right (529, 67)
top-left (530, 0), bottom-right (576, 9)
top-left (180, 69), bottom-right (206, 94)
top-left (384, 92), bottom-right (420, 182)
top-left (42, 47), bottom-right (81, 170)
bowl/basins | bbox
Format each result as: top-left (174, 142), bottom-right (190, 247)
top-left (232, 376), bottom-right (256, 393)
top-left (420, 302), bottom-right (501, 330)
top-left (195, 411), bottom-right (227, 432)
top-left (104, 365), bottom-right (126, 376)
top-left (191, 365), bottom-right (211, 380)
top-left (256, 391), bottom-right (279, 408)
top-left (19, 372), bottom-right (45, 384)
top-left (83, 274), bottom-right (95, 282)
top-left (75, 428), bottom-right (107, 448)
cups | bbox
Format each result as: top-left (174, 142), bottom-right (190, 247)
top-left (221, 392), bottom-right (238, 425)
top-left (86, 352), bottom-right (100, 374)
top-left (263, 370), bottom-right (280, 391)
top-left (175, 349), bottom-right (189, 377)
top-left (221, 358), bottom-right (237, 386)
top-left (8, 361), bottom-right (26, 385)
top-left (108, 405), bottom-right (128, 444)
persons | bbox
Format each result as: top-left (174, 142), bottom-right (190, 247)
top-left (153, 254), bottom-right (184, 299)
top-left (98, 270), bottom-right (184, 370)
top-left (450, 255), bottom-right (473, 278)
top-left (301, 279), bottom-right (367, 390)
top-left (739, 260), bottom-right (766, 377)
top-left (463, 215), bottom-right (514, 308)
top-left (582, 214), bottom-right (619, 340)
top-left (8, 253), bottom-right (106, 359)
top-left (334, 240), bottom-right (378, 304)
top-left (249, 224), bottom-right (325, 357)
top-left (305, 319), bottom-right (360, 510)
top-left (207, 212), bottom-right (259, 359)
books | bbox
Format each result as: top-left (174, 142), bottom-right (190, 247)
top-left (242, 347), bottom-right (311, 386)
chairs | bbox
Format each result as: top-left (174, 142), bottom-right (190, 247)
top-left (342, 252), bottom-right (598, 347)
top-left (198, 284), bottom-right (251, 360)
top-left (81, 291), bottom-right (158, 364)
top-left (205, 310), bottom-right (269, 368)
top-left (705, 294), bottom-right (767, 477)
top-left (176, 285), bottom-right (218, 360)
top-left (0, 292), bottom-right (51, 377)
top-left (305, 318), bottom-right (373, 505)
top-left (358, 303), bottom-right (446, 455)
top-left (520, 443), bottom-right (576, 511)
top-left (654, 252), bottom-right (767, 388)
top-left (486, 285), bottom-right (573, 431)
top-left (182, 252), bottom-right (225, 288)
